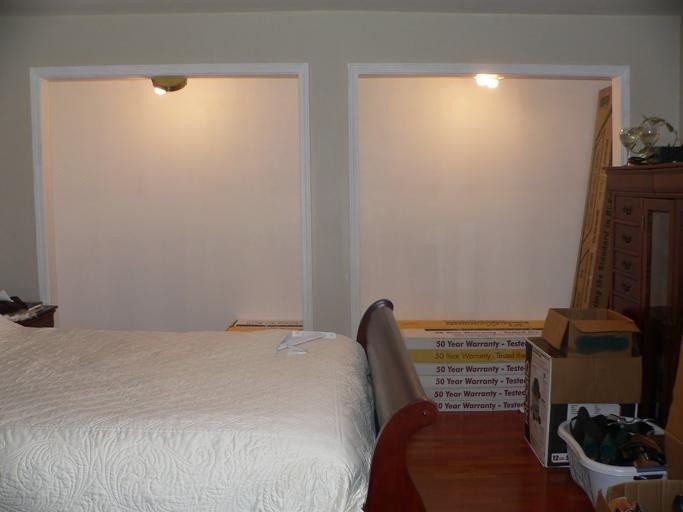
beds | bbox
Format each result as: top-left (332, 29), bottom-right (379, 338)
top-left (0, 297), bottom-right (440, 512)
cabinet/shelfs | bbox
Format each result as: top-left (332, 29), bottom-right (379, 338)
top-left (601, 165), bottom-right (683, 430)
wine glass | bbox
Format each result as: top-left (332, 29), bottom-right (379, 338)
top-left (638, 126), bottom-right (659, 153)
top-left (619, 127), bottom-right (638, 167)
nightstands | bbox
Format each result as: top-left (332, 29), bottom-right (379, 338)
top-left (1, 299), bottom-right (59, 328)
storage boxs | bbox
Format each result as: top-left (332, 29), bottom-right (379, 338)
top-left (522, 306), bottom-right (683, 512)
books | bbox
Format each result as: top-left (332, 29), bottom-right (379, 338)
top-left (23, 302), bottom-right (41, 311)
top-left (32, 305), bottom-right (44, 312)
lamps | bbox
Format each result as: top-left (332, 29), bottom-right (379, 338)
top-left (150, 75), bottom-right (188, 97)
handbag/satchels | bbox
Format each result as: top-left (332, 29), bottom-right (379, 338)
top-left (572, 407), bottom-right (665, 464)
